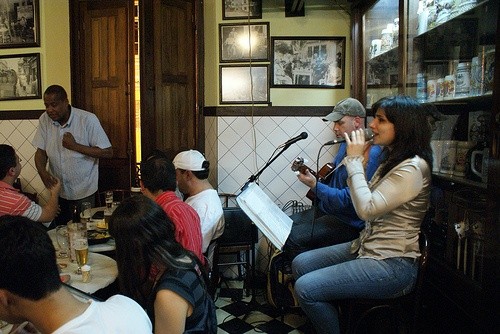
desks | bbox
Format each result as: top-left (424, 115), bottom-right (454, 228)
top-left (0, 204), bottom-right (140, 334)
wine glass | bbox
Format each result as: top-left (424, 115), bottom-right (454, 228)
top-left (56, 224), bottom-right (89, 275)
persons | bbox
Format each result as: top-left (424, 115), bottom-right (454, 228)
top-left (224, 28), bottom-right (238, 56)
top-left (0, 215), bottom-right (152, 334)
top-left (274, 50), bottom-right (342, 86)
top-left (19, 16), bottom-right (28, 42)
top-left (291, 96), bottom-right (434, 334)
top-left (32, 85), bottom-right (114, 228)
top-left (142, 158), bottom-right (205, 280)
top-left (172, 149), bottom-right (225, 254)
top-left (109, 197), bottom-right (219, 334)
top-left (0, 143), bottom-right (62, 221)
top-left (281, 98), bottom-right (381, 267)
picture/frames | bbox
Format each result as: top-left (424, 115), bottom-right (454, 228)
top-left (218, 64), bottom-right (271, 105)
top-left (221, 0), bottom-right (263, 21)
top-left (218, 22), bottom-right (270, 63)
top-left (270, 36), bottom-right (346, 90)
top-left (0, 0), bottom-right (41, 50)
top-left (0, 52), bottom-right (42, 100)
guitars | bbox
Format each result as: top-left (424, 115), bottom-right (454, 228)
top-left (292, 156), bottom-right (335, 202)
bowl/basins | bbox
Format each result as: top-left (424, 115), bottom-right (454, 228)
top-left (87, 229), bottom-right (112, 246)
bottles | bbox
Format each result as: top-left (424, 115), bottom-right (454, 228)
top-left (369, 17), bottom-right (400, 58)
top-left (81, 264), bottom-right (91, 283)
top-left (430, 140), bottom-right (474, 177)
top-left (415, 56), bottom-right (483, 103)
top-left (72, 205), bottom-right (81, 223)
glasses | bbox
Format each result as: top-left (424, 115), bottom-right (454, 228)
top-left (17, 158), bottom-right (22, 163)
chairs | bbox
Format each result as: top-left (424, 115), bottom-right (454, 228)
top-left (350, 227), bottom-right (433, 333)
top-left (215, 192), bottom-right (259, 297)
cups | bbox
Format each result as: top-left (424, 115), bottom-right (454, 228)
top-left (105, 191), bottom-right (113, 213)
top-left (81, 202), bottom-right (92, 223)
top-left (104, 209), bottom-right (112, 229)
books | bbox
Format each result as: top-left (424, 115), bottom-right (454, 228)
top-left (236, 182), bottom-right (293, 251)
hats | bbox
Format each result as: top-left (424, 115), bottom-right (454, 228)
top-left (173, 149), bottom-right (208, 171)
top-left (321, 98), bottom-right (366, 122)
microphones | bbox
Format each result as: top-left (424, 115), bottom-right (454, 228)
top-left (324, 128), bottom-right (374, 146)
top-left (277, 132), bottom-right (308, 149)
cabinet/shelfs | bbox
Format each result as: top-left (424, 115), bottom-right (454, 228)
top-left (345, 0), bottom-right (500, 334)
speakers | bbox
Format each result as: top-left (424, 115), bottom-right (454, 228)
top-left (208, 206), bottom-right (260, 246)
